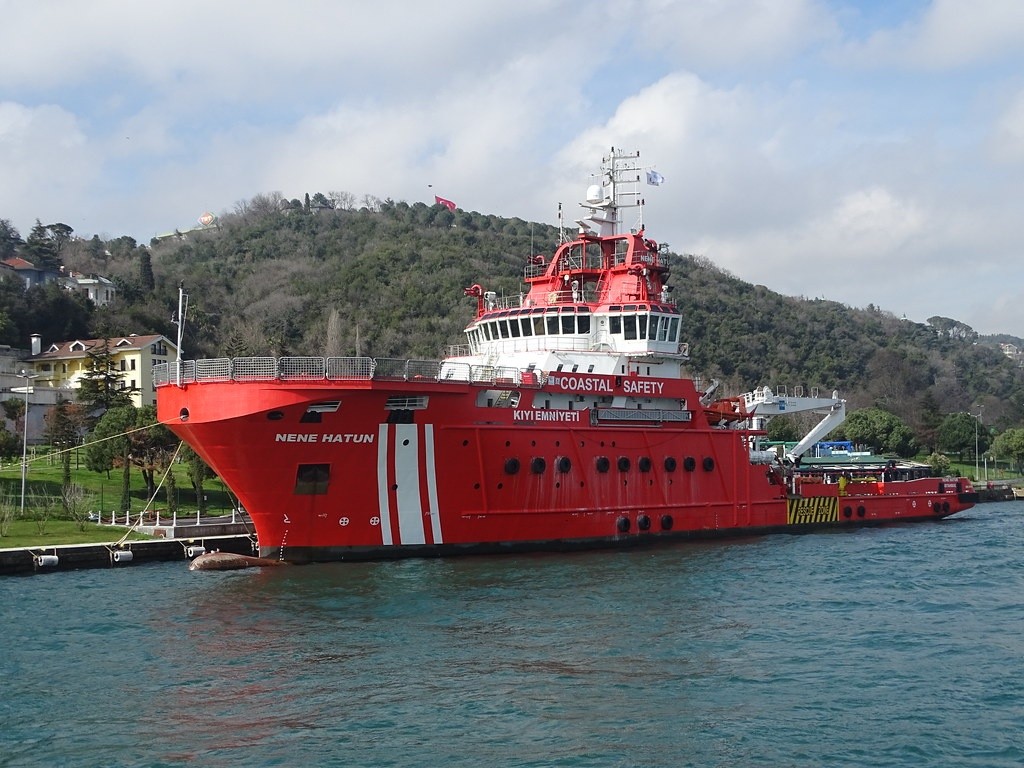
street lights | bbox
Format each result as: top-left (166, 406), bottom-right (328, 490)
top-left (16, 374), bottom-right (41, 516)
top-left (966, 412), bottom-right (983, 479)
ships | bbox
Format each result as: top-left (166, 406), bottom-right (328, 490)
top-left (152, 146), bottom-right (982, 568)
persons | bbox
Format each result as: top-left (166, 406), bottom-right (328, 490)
top-left (832, 387), bottom-right (840, 411)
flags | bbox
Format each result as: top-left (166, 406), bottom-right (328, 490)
top-left (646, 172), bottom-right (659, 185)
top-left (435, 197), bottom-right (456, 212)
top-left (651, 170), bottom-right (664, 183)
